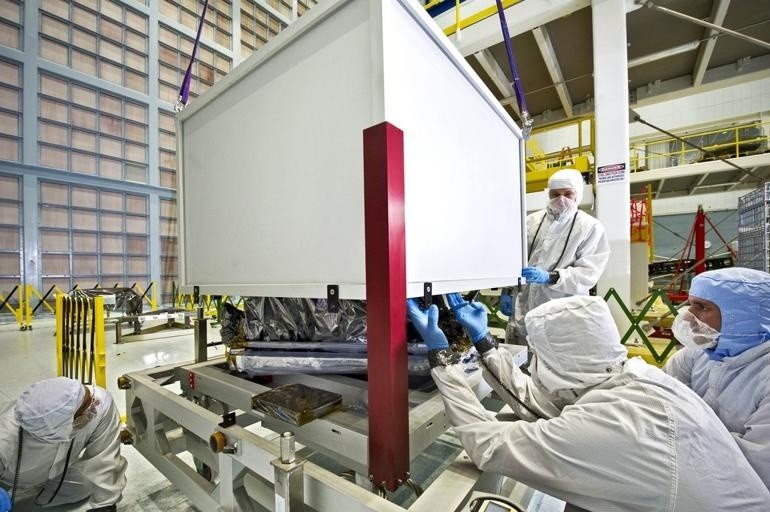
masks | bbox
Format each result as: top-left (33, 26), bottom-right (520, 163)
top-left (672, 310), bottom-right (721, 350)
top-left (527, 356), bottom-right (541, 389)
top-left (547, 196), bottom-right (573, 217)
top-left (74, 401), bottom-right (97, 427)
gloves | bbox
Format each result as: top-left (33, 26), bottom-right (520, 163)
top-left (523, 266), bottom-right (549, 284)
top-left (0, 489), bottom-right (12, 512)
top-left (407, 297), bottom-right (448, 349)
top-left (448, 294), bottom-right (490, 343)
top-left (500, 296), bottom-right (514, 314)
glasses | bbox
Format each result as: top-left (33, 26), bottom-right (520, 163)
top-left (80, 385), bottom-right (96, 413)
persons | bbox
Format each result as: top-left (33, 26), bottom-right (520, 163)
top-left (405, 291), bottom-right (770, 512)
top-left (517, 170), bottom-right (610, 346)
top-left (1, 376), bottom-right (127, 512)
top-left (672, 267), bottom-right (770, 491)
